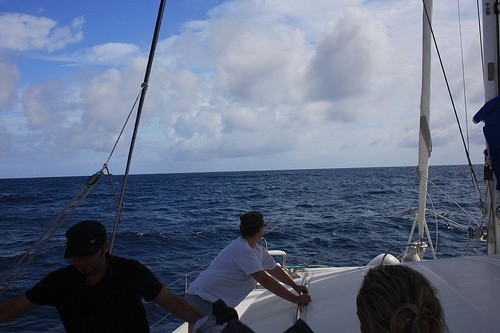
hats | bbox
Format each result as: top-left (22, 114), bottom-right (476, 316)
top-left (240, 212), bottom-right (264, 225)
top-left (64, 220), bottom-right (106, 258)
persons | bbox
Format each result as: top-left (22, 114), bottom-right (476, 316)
top-left (355, 263), bottom-right (450, 333)
top-left (181, 210), bottom-right (313, 333)
top-left (0, 219), bottom-right (205, 333)
